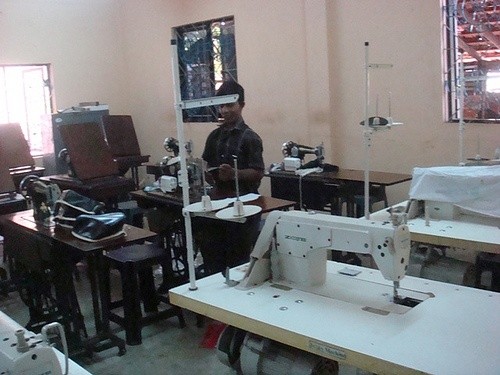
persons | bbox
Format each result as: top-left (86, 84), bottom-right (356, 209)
top-left (202, 81), bottom-right (264, 275)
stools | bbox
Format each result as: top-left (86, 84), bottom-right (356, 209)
top-left (101, 245), bottom-right (188, 347)
top-left (474, 251), bottom-right (499, 295)
top-left (342, 193), bottom-right (388, 219)
top-left (116, 200), bottom-right (144, 228)
